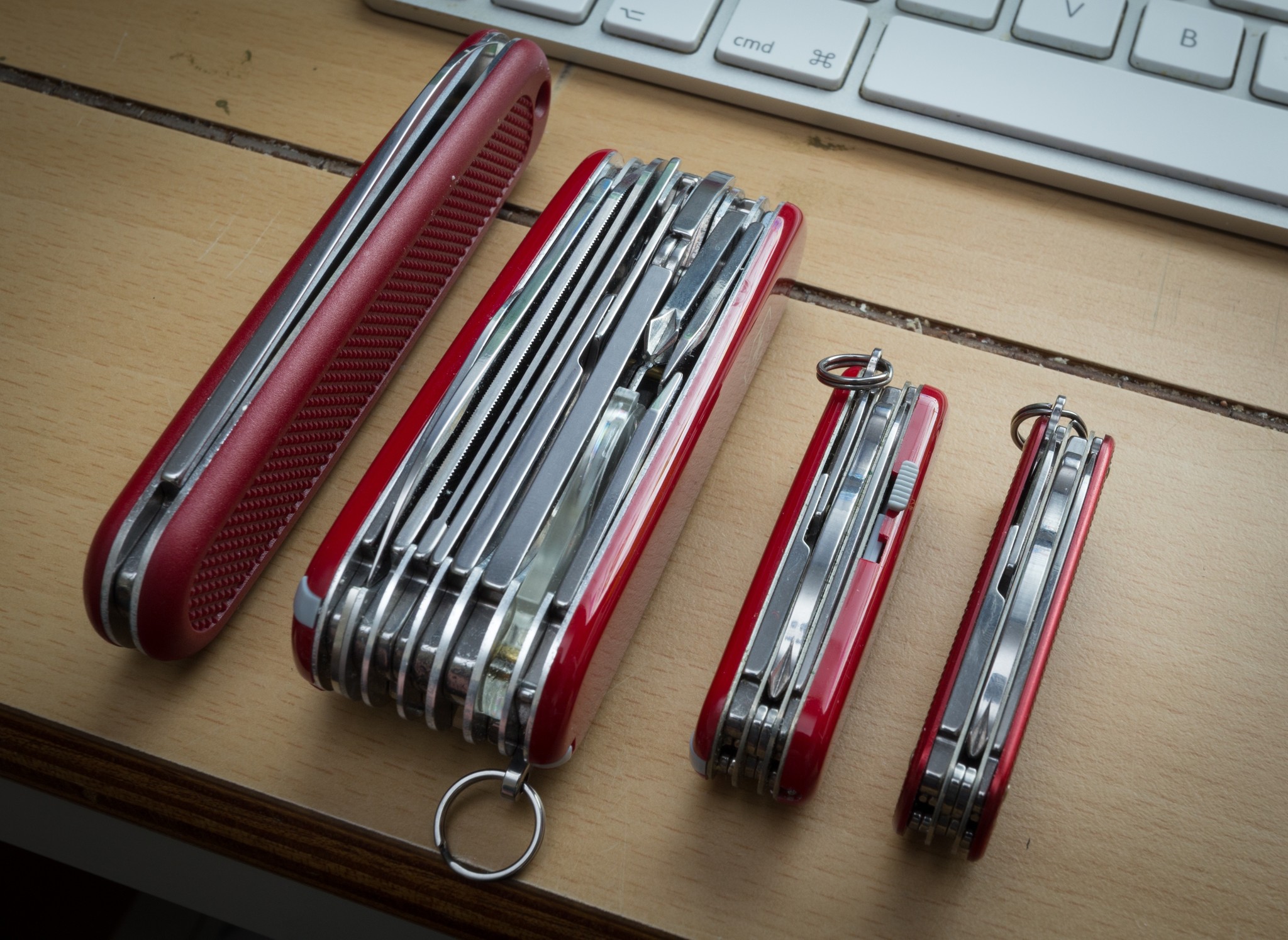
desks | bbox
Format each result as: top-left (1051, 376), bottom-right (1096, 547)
top-left (3, 0), bottom-right (1285, 939)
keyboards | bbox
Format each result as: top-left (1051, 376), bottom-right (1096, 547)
top-left (354, 2), bottom-right (1288, 248)
